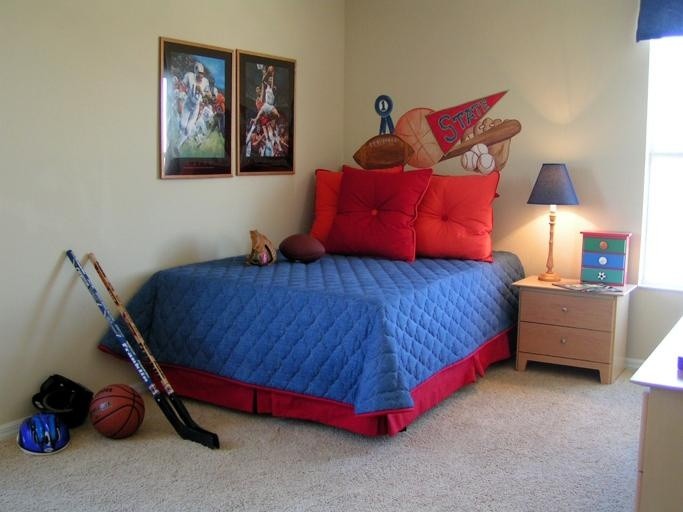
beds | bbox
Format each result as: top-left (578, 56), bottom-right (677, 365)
top-left (93, 250), bottom-right (524, 439)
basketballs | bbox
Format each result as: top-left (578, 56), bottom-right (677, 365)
top-left (393, 108), bottom-right (444, 168)
top-left (267, 66), bottom-right (274, 76)
top-left (92, 384), bottom-right (144, 439)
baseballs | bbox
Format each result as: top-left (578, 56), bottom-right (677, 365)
top-left (462, 144), bottom-right (495, 173)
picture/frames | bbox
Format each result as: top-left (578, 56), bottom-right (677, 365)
top-left (237, 46), bottom-right (298, 177)
top-left (155, 34), bottom-right (237, 184)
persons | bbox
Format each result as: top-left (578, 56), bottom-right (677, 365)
top-left (168, 62), bottom-right (223, 158)
top-left (246, 63), bottom-right (287, 158)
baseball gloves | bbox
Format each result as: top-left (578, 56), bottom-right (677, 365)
top-left (461, 117), bottom-right (511, 174)
top-left (247, 231), bottom-right (277, 264)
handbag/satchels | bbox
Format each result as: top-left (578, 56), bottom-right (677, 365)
top-left (31, 374), bottom-right (93, 429)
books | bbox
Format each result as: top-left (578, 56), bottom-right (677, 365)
top-left (552, 281), bottom-right (607, 293)
top-left (590, 282), bottom-right (622, 292)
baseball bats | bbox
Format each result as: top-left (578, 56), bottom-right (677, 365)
top-left (440, 120), bottom-right (521, 162)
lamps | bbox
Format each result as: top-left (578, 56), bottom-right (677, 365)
top-left (526, 163), bottom-right (581, 284)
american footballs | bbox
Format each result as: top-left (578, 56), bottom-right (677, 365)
top-left (352, 134), bottom-right (414, 169)
top-left (279, 233), bottom-right (325, 262)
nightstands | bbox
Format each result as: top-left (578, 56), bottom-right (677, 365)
top-left (508, 273), bottom-right (639, 387)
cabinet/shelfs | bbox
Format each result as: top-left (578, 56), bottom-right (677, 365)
top-left (576, 229), bottom-right (634, 288)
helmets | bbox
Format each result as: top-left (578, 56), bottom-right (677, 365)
top-left (17, 414), bottom-right (70, 456)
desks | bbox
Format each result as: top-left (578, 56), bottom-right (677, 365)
top-left (629, 305), bottom-right (683, 512)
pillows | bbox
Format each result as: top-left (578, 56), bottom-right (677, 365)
top-left (412, 170), bottom-right (502, 265)
top-left (309, 164), bottom-right (406, 248)
top-left (322, 165), bottom-right (433, 262)
